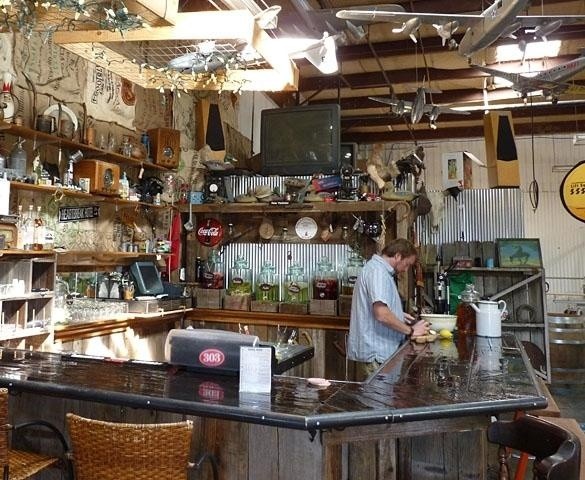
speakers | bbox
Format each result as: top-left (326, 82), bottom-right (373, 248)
top-left (195, 97), bottom-right (226, 162)
top-left (484, 110), bottom-right (521, 189)
top-left (146, 127), bottom-right (181, 168)
top-left (73, 159), bottom-right (121, 196)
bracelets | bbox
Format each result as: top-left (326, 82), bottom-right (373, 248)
top-left (410, 327), bottom-right (414, 336)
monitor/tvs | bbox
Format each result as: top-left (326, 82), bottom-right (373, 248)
top-left (130, 262), bottom-right (165, 294)
top-left (260, 103), bottom-right (340, 176)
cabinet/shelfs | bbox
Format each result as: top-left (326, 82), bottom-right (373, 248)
top-left (0, 122), bottom-right (187, 348)
top-left (191, 196), bottom-right (408, 328)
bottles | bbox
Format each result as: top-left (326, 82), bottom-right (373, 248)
top-left (95, 119), bottom-right (119, 150)
top-left (16, 205), bottom-right (43, 251)
top-left (0, 136), bottom-right (27, 177)
top-left (458, 283), bottom-right (479, 332)
top-left (204, 220), bottom-right (211, 244)
top-left (196, 249), bottom-right (363, 300)
top-left (224, 222), bottom-right (348, 245)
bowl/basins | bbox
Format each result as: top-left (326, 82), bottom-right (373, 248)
top-left (426, 341), bottom-right (458, 359)
top-left (422, 313), bottom-right (458, 332)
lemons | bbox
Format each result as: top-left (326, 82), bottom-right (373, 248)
top-left (440, 339), bottom-right (450, 347)
top-left (440, 329), bottom-right (453, 338)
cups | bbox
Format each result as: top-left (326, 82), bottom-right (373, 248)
top-left (36, 115), bottom-right (57, 134)
top-left (69, 299), bottom-right (127, 320)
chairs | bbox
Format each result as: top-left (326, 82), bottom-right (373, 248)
top-left (0, 389), bottom-right (219, 478)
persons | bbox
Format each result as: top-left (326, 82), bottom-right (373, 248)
top-left (347, 238), bottom-right (431, 383)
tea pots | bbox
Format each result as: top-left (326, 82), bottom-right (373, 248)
top-left (473, 340), bottom-right (507, 376)
top-left (468, 296), bottom-right (505, 339)
